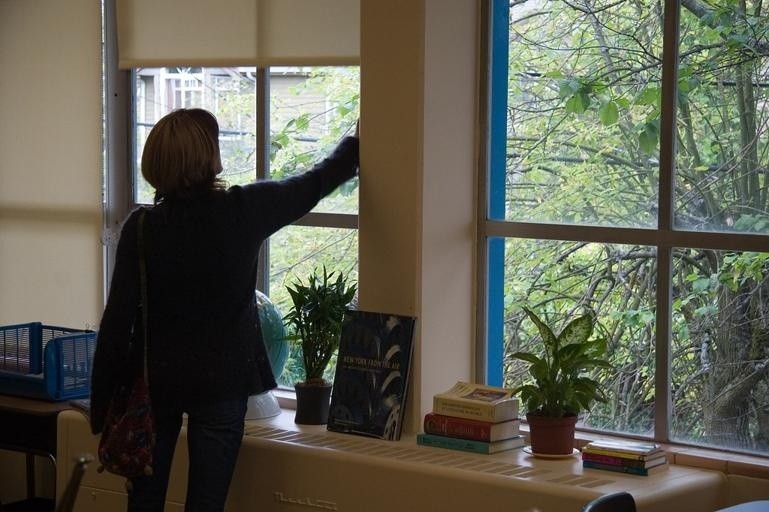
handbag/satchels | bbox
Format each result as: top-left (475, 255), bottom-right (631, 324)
top-left (98, 375), bottom-right (158, 480)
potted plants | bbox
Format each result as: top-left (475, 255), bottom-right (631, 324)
top-left (276, 264), bottom-right (357, 425)
top-left (507, 306), bottom-right (617, 455)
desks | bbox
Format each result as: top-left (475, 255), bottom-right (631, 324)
top-left (0, 394), bottom-right (76, 501)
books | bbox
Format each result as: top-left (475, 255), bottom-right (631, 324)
top-left (581, 441), bottom-right (669, 476)
top-left (417, 380), bottom-right (525, 454)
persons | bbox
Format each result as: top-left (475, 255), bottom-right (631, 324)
top-left (90, 108), bottom-right (360, 511)
